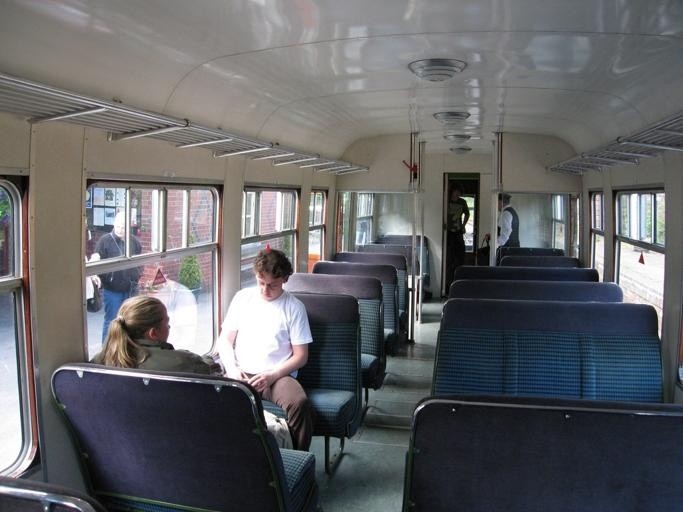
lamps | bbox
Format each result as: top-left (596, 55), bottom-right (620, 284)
top-left (409, 58), bottom-right (466, 81)
top-left (444, 134), bottom-right (470, 144)
top-left (434, 112), bottom-right (470, 126)
top-left (449, 148), bottom-right (472, 155)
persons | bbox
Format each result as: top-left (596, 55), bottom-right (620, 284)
top-left (87, 295), bottom-right (211, 378)
top-left (216, 248), bottom-right (313, 451)
top-left (88, 211), bottom-right (142, 347)
top-left (485, 193), bottom-right (520, 259)
top-left (447, 187), bottom-right (470, 278)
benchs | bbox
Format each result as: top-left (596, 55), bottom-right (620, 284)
top-left (262, 290), bottom-right (362, 473)
top-left (403, 247), bottom-right (683, 512)
top-left (283, 270), bottom-right (386, 416)
top-left (48, 361), bottom-right (318, 510)
top-left (311, 235), bottom-right (429, 357)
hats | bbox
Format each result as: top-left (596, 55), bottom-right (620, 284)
top-left (498, 192), bottom-right (512, 200)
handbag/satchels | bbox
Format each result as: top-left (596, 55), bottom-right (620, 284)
top-left (475, 235), bottom-right (490, 267)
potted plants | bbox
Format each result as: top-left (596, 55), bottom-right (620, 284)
top-left (180, 234), bottom-right (202, 305)
